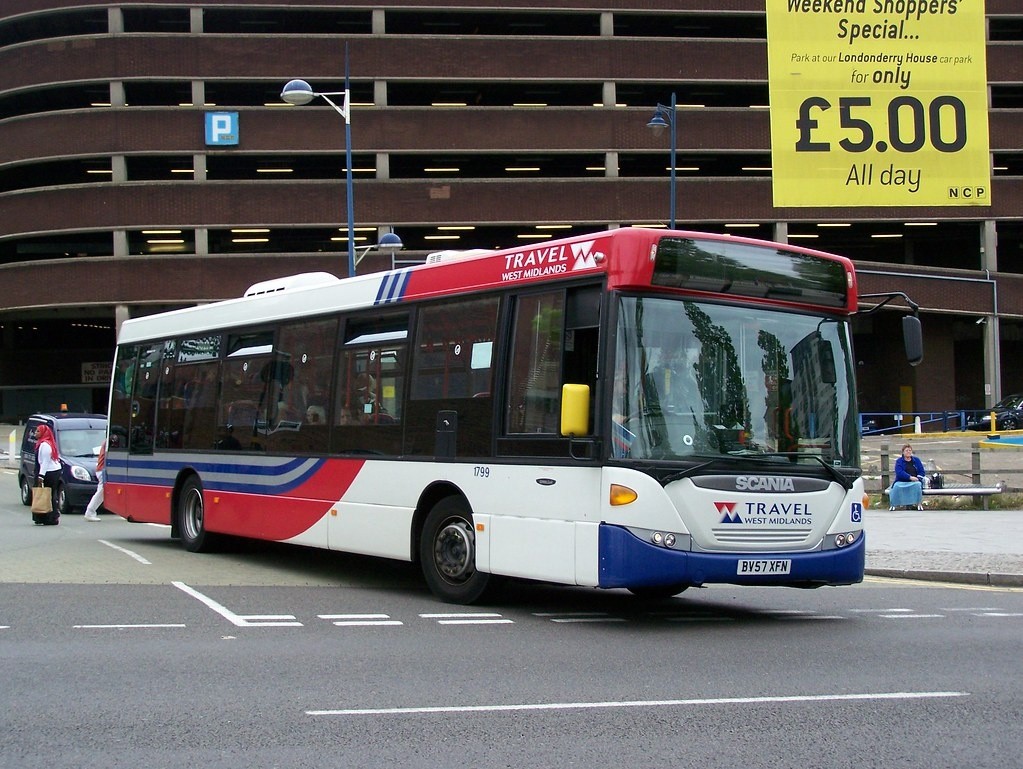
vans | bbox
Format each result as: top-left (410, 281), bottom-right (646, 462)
top-left (17, 413), bottom-right (107, 513)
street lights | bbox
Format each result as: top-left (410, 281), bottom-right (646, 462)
top-left (279, 40), bottom-right (405, 279)
top-left (644, 93), bottom-right (677, 230)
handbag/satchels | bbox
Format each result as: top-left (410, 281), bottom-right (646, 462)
top-left (926, 473), bottom-right (944, 489)
top-left (31, 483), bottom-right (53, 514)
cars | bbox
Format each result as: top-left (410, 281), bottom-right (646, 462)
top-left (966, 394), bottom-right (1023, 431)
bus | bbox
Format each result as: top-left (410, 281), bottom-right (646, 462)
top-left (100, 228), bottom-right (927, 607)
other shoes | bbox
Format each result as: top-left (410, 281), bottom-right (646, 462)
top-left (889, 506), bottom-right (895, 511)
top-left (83, 516), bottom-right (101, 522)
top-left (917, 506), bottom-right (923, 511)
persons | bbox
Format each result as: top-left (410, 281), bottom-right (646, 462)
top-left (349, 373), bottom-right (393, 425)
top-left (31, 425), bottom-right (64, 524)
top-left (887, 444), bottom-right (926, 511)
top-left (84, 440), bottom-right (107, 521)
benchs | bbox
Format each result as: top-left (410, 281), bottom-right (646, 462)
top-left (885, 482), bottom-right (1007, 511)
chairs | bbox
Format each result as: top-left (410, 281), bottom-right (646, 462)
top-left (120, 369), bottom-right (401, 426)
